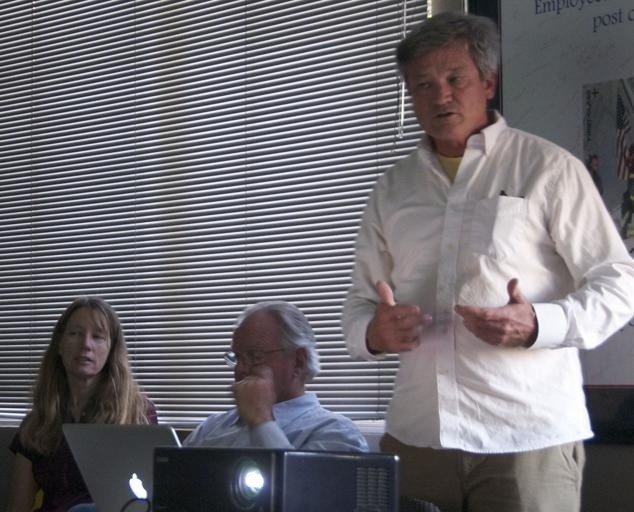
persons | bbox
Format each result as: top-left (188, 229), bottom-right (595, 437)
top-left (9, 296), bottom-right (159, 511)
top-left (183, 301), bottom-right (368, 452)
top-left (340, 12), bottom-right (634, 511)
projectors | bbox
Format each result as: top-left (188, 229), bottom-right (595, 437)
top-left (152, 445), bottom-right (399, 511)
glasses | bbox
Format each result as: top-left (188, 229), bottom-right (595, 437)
top-left (224, 348), bottom-right (285, 366)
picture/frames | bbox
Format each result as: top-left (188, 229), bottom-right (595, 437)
top-left (464, 0), bottom-right (634, 448)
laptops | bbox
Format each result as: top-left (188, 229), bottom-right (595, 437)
top-left (63, 422), bottom-right (181, 511)
